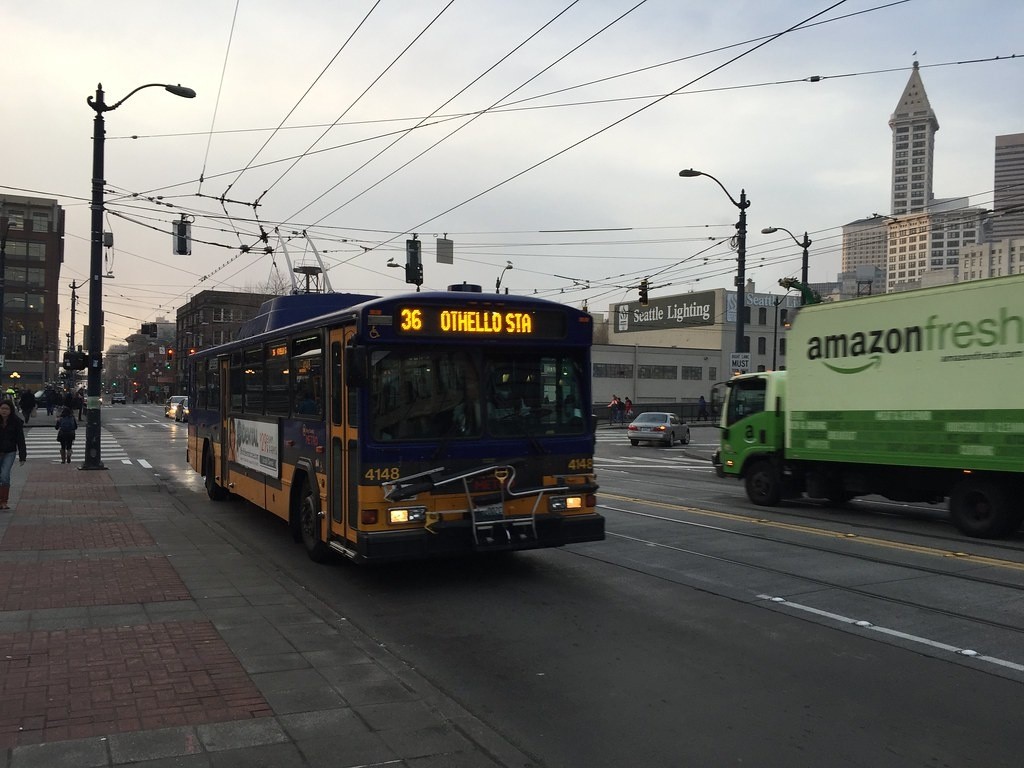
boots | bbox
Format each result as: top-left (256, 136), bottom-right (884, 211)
top-left (66, 449), bottom-right (72, 464)
top-left (0, 485), bottom-right (10, 509)
top-left (60, 450), bottom-right (66, 464)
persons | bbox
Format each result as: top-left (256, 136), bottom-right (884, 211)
top-left (55, 407), bottom-right (78, 464)
top-left (0, 400), bottom-right (27, 509)
top-left (45, 386), bottom-right (87, 421)
top-left (0, 383), bottom-right (35, 423)
top-left (696, 396), bottom-right (707, 421)
top-left (607, 394), bottom-right (632, 422)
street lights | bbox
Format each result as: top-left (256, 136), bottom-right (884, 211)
top-left (386, 263), bottom-right (421, 292)
top-left (761, 226), bottom-right (813, 305)
top-left (74, 82), bottom-right (196, 471)
top-left (678, 167), bottom-right (751, 377)
top-left (496, 265), bottom-right (514, 294)
top-left (772, 287), bottom-right (801, 371)
top-left (66, 274), bottom-right (115, 392)
top-left (9, 372), bottom-right (21, 393)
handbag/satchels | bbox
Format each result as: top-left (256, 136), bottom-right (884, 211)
top-left (31, 407), bottom-right (37, 418)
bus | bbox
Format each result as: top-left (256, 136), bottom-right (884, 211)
top-left (187, 226), bottom-right (607, 567)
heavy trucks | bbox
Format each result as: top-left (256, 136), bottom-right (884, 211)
top-left (709, 274), bottom-right (1024, 540)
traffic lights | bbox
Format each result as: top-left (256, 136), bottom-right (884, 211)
top-left (166, 348), bottom-right (173, 361)
top-left (638, 281), bottom-right (648, 307)
top-left (133, 389), bottom-right (139, 394)
top-left (166, 362), bottom-right (172, 369)
top-left (133, 363), bottom-right (137, 371)
top-left (132, 381), bottom-right (137, 386)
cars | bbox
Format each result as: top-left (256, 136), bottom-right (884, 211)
top-left (165, 396), bottom-right (188, 420)
top-left (175, 398), bottom-right (189, 423)
top-left (78, 380), bottom-right (103, 406)
top-left (33, 389), bottom-right (67, 410)
top-left (112, 393), bottom-right (126, 405)
top-left (627, 412), bottom-right (690, 447)
